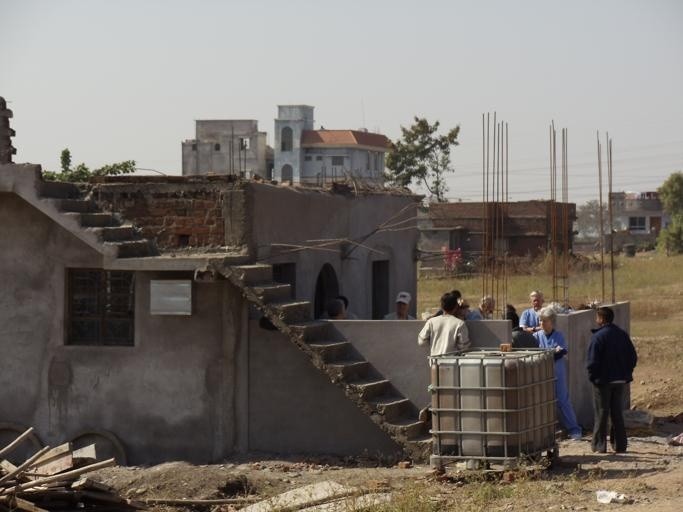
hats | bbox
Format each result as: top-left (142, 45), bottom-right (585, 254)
top-left (395, 291), bottom-right (412, 305)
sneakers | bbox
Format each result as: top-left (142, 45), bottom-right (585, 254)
top-left (572, 427), bottom-right (582, 440)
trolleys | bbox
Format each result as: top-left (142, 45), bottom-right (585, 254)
top-left (427, 348), bottom-right (560, 476)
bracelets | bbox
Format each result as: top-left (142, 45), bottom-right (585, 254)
top-left (531, 326), bottom-right (535, 332)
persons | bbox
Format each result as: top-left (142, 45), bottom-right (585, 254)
top-left (426, 289), bottom-right (463, 320)
top-left (500, 303), bottom-right (538, 348)
top-left (532, 306), bottom-right (581, 439)
top-left (463, 295), bottom-right (493, 319)
top-left (326, 298), bottom-right (346, 320)
top-left (585, 307), bottom-right (637, 452)
top-left (318, 295), bottom-right (360, 319)
top-left (381, 292), bottom-right (415, 320)
top-left (518, 291), bottom-right (545, 336)
top-left (417, 293), bottom-right (471, 423)
